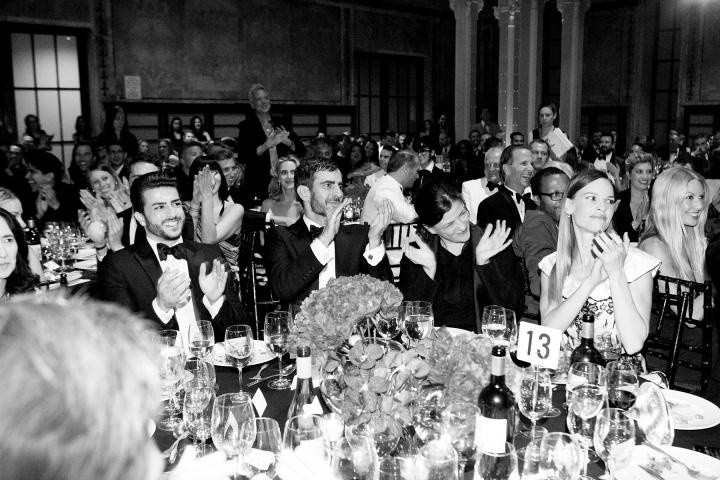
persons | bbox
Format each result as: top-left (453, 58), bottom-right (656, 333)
top-left (2, 103), bottom-right (720, 400)
top-left (234, 84), bottom-right (306, 189)
top-left (0, 293), bottom-right (183, 480)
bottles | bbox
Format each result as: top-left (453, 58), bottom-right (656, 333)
top-left (24, 217), bottom-right (43, 266)
top-left (342, 197), bottom-right (365, 221)
top-left (60, 274), bottom-right (68, 288)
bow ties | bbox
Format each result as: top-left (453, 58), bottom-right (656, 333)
top-left (598, 155), bottom-right (607, 160)
top-left (154, 242), bottom-right (190, 262)
top-left (485, 182), bottom-right (501, 193)
top-left (307, 223), bottom-right (328, 241)
top-left (513, 191), bottom-right (532, 204)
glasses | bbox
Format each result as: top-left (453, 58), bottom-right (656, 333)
top-left (537, 189), bottom-right (565, 201)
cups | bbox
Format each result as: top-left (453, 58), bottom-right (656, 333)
top-left (442, 162), bottom-right (450, 172)
top-left (655, 156), bottom-right (672, 174)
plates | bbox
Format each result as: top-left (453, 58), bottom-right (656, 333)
top-left (655, 388), bottom-right (720, 431)
top-left (609, 444), bottom-right (720, 480)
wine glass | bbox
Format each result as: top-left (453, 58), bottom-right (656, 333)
top-left (41, 221), bottom-right (84, 272)
top-left (146, 300), bottom-right (640, 480)
